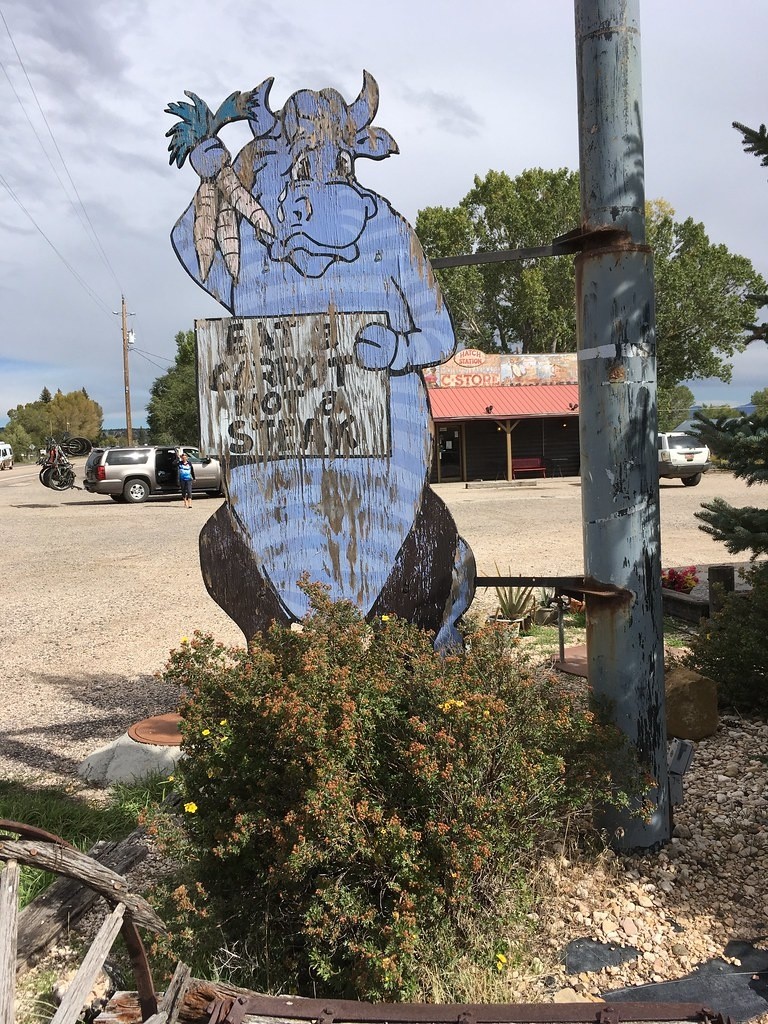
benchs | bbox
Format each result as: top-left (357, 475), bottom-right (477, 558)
top-left (549, 457), bottom-right (581, 478)
top-left (512, 458), bottom-right (547, 480)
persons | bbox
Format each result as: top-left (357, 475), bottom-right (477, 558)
top-left (172, 453), bottom-right (196, 509)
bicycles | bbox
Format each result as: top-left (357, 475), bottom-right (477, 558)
top-left (35, 432), bottom-right (92, 492)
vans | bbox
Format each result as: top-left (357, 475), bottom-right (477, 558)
top-left (0, 441), bottom-right (15, 471)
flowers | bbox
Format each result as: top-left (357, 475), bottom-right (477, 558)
top-left (661, 565), bottom-right (701, 592)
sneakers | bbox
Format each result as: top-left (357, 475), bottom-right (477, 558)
top-left (184, 504), bottom-right (187, 508)
top-left (189, 506), bottom-right (193, 508)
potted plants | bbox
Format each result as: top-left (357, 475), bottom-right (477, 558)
top-left (520, 605), bottom-right (532, 631)
top-left (534, 586), bottom-right (554, 624)
top-left (480, 560), bottom-right (540, 641)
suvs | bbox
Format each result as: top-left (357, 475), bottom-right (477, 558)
top-left (657, 430), bottom-right (711, 487)
top-left (85, 444), bottom-right (228, 503)
